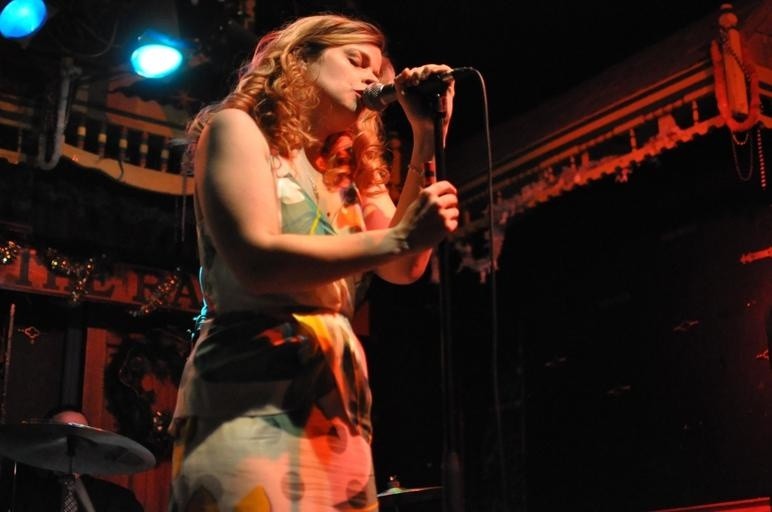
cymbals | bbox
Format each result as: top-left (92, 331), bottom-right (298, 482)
top-left (376, 484), bottom-right (442, 498)
top-left (0, 420), bottom-right (159, 475)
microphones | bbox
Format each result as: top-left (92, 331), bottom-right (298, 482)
top-left (363, 65), bottom-right (471, 112)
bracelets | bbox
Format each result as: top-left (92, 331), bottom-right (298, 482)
top-left (407, 164), bottom-right (426, 177)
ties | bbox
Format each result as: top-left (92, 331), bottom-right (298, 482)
top-left (63, 479), bottom-right (77, 512)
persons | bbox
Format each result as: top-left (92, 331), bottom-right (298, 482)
top-left (1, 405), bottom-right (141, 512)
top-left (166, 13), bottom-right (459, 511)
